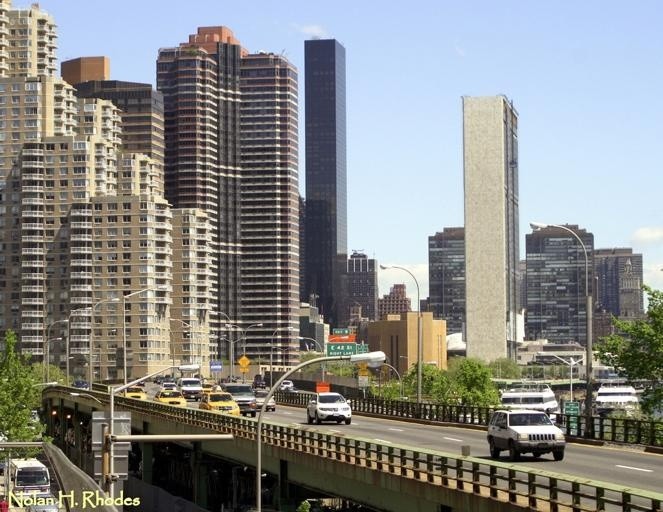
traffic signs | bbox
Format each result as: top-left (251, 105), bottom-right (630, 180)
top-left (356, 345), bottom-right (369, 354)
top-left (332, 328), bottom-right (351, 335)
top-left (326, 342), bottom-right (357, 360)
top-left (563, 400), bottom-right (583, 433)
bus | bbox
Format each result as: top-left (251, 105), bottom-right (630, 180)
top-left (10, 456), bottom-right (52, 504)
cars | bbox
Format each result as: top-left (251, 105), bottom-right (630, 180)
top-left (154, 374), bottom-right (294, 419)
top-left (0, 409), bottom-right (41, 452)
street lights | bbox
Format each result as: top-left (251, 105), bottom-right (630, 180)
top-left (109, 364), bottom-right (201, 511)
top-left (535, 348), bottom-right (600, 435)
top-left (167, 309), bottom-right (348, 395)
top-left (43, 283), bottom-right (173, 402)
top-left (254, 350), bottom-right (387, 512)
top-left (4, 381), bottom-right (58, 400)
top-left (529, 221), bottom-right (594, 437)
top-left (380, 264), bottom-right (421, 406)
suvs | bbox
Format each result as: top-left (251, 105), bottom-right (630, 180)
top-left (486, 405), bottom-right (566, 462)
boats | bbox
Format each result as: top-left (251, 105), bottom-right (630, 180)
top-left (595, 376), bottom-right (640, 422)
top-left (489, 381), bottom-right (562, 428)
